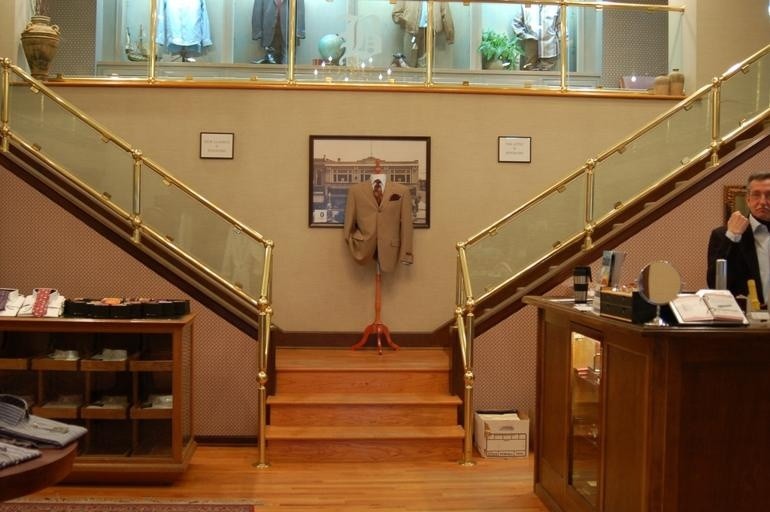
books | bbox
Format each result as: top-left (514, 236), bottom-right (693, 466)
top-left (667, 288), bottom-right (749, 327)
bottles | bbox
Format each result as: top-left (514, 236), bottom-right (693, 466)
top-left (745, 279), bottom-right (761, 313)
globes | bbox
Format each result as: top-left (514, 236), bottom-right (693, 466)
top-left (319, 34), bottom-right (346, 66)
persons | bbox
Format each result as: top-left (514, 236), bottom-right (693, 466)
top-left (706, 170), bottom-right (770, 312)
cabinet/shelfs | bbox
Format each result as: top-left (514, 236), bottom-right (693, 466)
top-left (0, 311), bottom-right (200, 487)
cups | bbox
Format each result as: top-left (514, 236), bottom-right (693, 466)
top-left (573, 266), bottom-right (593, 304)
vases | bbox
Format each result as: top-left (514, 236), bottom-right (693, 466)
top-left (654, 70), bottom-right (670, 97)
top-left (21, 15), bottom-right (62, 80)
top-left (669, 67), bottom-right (685, 96)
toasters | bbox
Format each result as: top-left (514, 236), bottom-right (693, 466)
top-left (612, 284), bottom-right (619, 293)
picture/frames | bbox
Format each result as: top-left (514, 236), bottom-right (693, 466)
top-left (200, 131), bottom-right (235, 160)
top-left (308, 134), bottom-right (432, 230)
top-left (721, 183), bottom-right (751, 226)
top-left (497, 135), bottom-right (533, 164)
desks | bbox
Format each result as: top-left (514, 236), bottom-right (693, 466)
top-left (520, 294), bottom-right (769, 511)
top-left (0, 438), bottom-right (79, 504)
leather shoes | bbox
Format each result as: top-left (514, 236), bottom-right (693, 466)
top-left (266, 54), bottom-right (275, 63)
top-left (396, 58), bottom-right (409, 67)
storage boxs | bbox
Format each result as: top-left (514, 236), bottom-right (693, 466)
top-left (473, 407), bottom-right (531, 459)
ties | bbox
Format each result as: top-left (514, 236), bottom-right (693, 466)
top-left (33, 288), bottom-right (51, 318)
top-left (373, 179), bottom-right (383, 206)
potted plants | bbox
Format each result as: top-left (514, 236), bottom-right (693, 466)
top-left (476, 27), bottom-right (529, 71)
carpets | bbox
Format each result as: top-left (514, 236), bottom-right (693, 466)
top-left (0, 493), bottom-right (254, 511)
